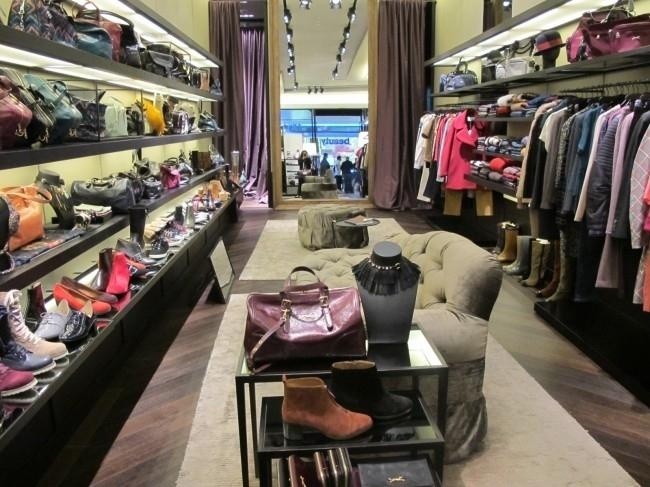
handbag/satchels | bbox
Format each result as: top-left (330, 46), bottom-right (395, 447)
top-left (71, 170), bottom-right (163, 215)
top-left (165, 99), bottom-right (220, 135)
top-left (245, 266), bottom-right (369, 378)
top-left (0, 67), bottom-right (143, 150)
top-left (159, 143), bottom-right (225, 188)
top-left (9, 0), bottom-right (147, 68)
top-left (494, 45), bottom-right (536, 79)
top-left (608, 12), bottom-right (650, 55)
top-left (445, 62), bottom-right (478, 90)
top-left (2, 180), bottom-right (53, 252)
top-left (564, 9), bottom-right (635, 63)
top-left (149, 39), bottom-right (222, 98)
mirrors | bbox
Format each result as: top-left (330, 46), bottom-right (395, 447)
top-left (272, 1), bottom-right (375, 204)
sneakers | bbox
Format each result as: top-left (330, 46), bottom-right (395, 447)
top-left (149, 221), bottom-right (194, 259)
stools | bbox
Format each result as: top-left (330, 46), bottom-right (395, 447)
top-left (295, 202), bottom-right (371, 252)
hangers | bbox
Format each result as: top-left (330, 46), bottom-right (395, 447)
top-left (559, 79), bottom-right (649, 119)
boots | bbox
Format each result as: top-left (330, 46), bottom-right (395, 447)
top-left (279, 372), bottom-right (376, 440)
top-left (0, 306), bottom-right (57, 377)
top-left (0, 287), bottom-right (68, 361)
top-left (88, 247), bottom-right (112, 292)
top-left (184, 206), bottom-right (195, 227)
top-left (331, 357), bottom-right (413, 427)
top-left (107, 251), bottom-right (130, 295)
top-left (24, 282), bottom-right (47, 332)
top-left (128, 205), bottom-right (147, 245)
top-left (489, 221), bottom-right (582, 304)
top-left (0, 361), bottom-right (39, 400)
top-left (175, 206), bottom-right (184, 226)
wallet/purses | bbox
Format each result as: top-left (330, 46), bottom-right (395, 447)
top-left (270, 446), bottom-right (436, 487)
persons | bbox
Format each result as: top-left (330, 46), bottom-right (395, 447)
top-left (340, 156), bottom-right (356, 194)
top-left (332, 155), bottom-right (342, 191)
top-left (293, 149), bottom-right (300, 160)
top-left (295, 150), bottom-right (312, 198)
top-left (320, 152), bottom-right (330, 176)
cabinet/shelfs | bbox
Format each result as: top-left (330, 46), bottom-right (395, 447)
top-left (233, 318), bottom-right (449, 487)
top-left (0, 0), bottom-right (240, 456)
top-left (422, 0), bottom-right (650, 413)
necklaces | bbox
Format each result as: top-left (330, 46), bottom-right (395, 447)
top-left (368, 256), bottom-right (402, 271)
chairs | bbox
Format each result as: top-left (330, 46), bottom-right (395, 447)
top-left (288, 227), bottom-right (505, 464)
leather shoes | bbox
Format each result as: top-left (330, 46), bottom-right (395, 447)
top-left (117, 237), bottom-right (156, 277)
top-left (33, 276), bottom-right (118, 342)
top-left (191, 190), bottom-right (222, 212)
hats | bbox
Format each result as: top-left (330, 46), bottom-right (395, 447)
top-left (532, 29), bottom-right (566, 56)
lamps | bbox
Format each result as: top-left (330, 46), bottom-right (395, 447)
top-left (279, 0), bottom-right (357, 95)
